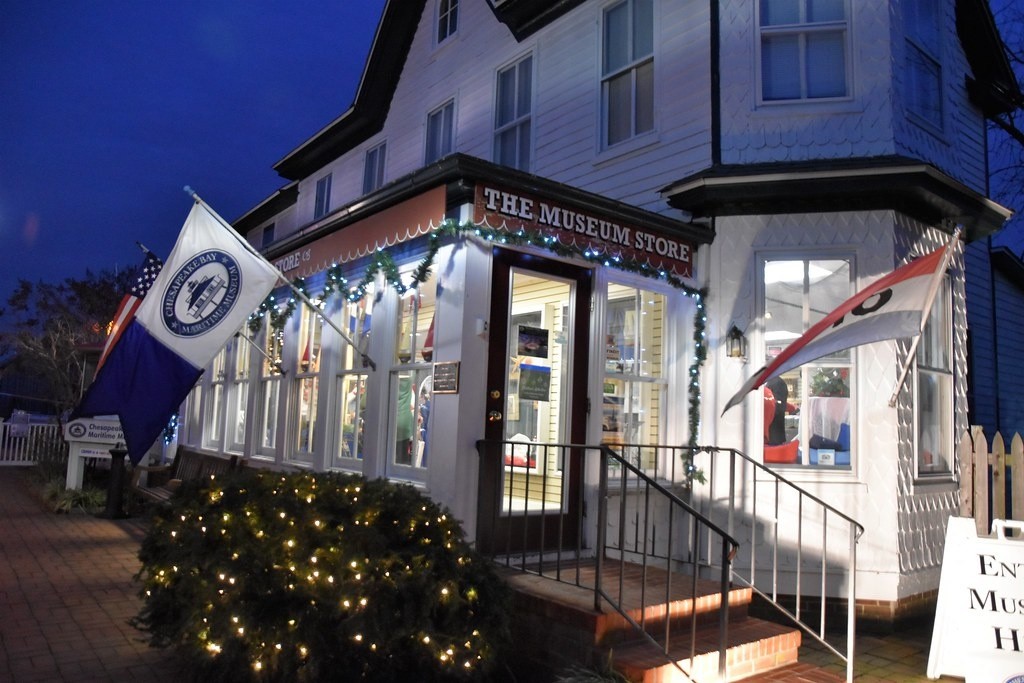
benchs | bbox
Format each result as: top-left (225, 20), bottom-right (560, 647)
top-left (132, 445), bottom-right (238, 517)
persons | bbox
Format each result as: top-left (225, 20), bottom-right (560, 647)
top-left (763, 356), bottom-right (789, 446)
top-left (396, 368), bottom-right (416, 463)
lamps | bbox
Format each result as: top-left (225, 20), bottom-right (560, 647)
top-left (725, 325), bottom-right (746, 357)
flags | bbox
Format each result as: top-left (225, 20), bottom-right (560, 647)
top-left (721, 241), bottom-right (947, 417)
top-left (94, 251), bottom-right (164, 381)
